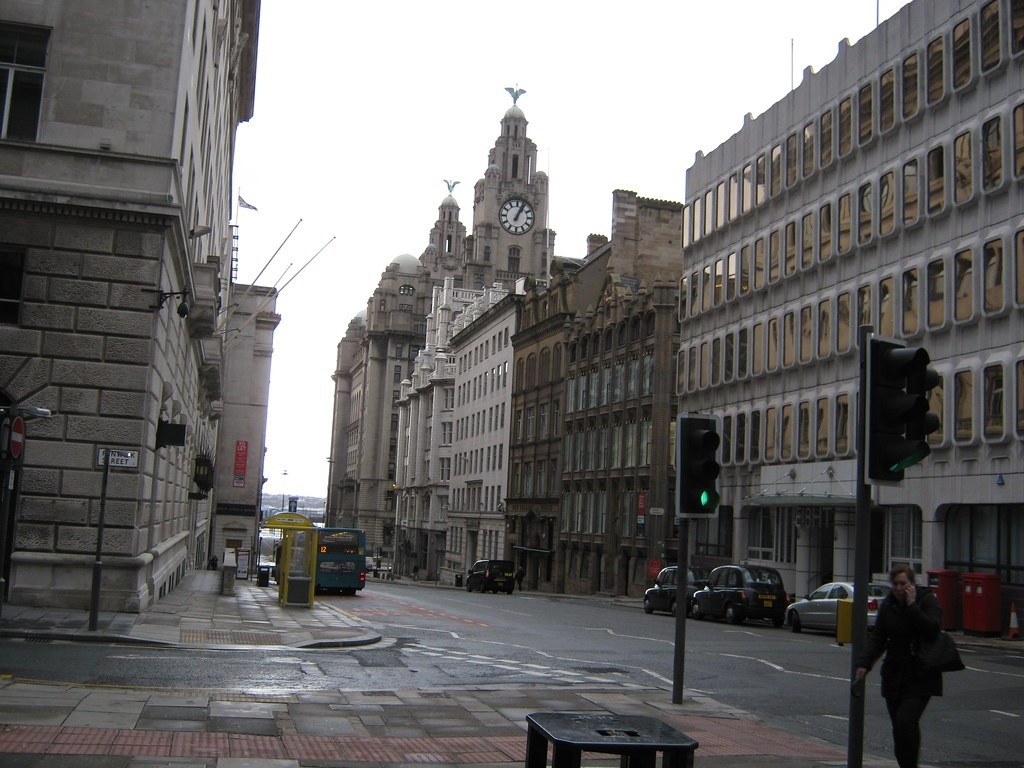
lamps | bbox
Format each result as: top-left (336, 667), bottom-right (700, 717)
top-left (189, 223), bottom-right (213, 241)
top-left (141, 283), bottom-right (192, 318)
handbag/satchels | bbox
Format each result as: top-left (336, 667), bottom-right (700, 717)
top-left (903, 629), bottom-right (966, 675)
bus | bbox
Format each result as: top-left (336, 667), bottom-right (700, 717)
top-left (274, 527), bottom-right (367, 596)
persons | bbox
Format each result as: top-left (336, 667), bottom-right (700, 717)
top-left (852, 563), bottom-right (943, 768)
top-left (514, 567), bottom-right (526, 592)
top-left (413, 565), bottom-right (420, 581)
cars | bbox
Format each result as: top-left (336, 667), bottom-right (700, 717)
top-left (643, 565), bottom-right (708, 618)
top-left (690, 563), bottom-right (787, 629)
top-left (465, 559), bottom-right (517, 594)
top-left (783, 581), bottom-right (894, 634)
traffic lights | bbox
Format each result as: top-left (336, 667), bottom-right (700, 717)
top-left (676, 412), bottom-right (722, 518)
top-left (865, 332), bottom-right (931, 484)
top-left (904, 362), bottom-right (940, 445)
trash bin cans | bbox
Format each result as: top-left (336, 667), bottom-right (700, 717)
top-left (454, 574), bottom-right (463, 587)
top-left (257, 566), bottom-right (270, 587)
top-left (373, 569), bottom-right (378, 578)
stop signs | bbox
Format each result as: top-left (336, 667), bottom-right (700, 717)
top-left (10, 416), bottom-right (24, 458)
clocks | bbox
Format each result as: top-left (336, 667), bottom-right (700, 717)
top-left (499, 198), bottom-right (535, 236)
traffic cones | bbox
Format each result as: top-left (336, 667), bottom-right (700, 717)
top-left (1000, 601), bottom-right (1024, 641)
